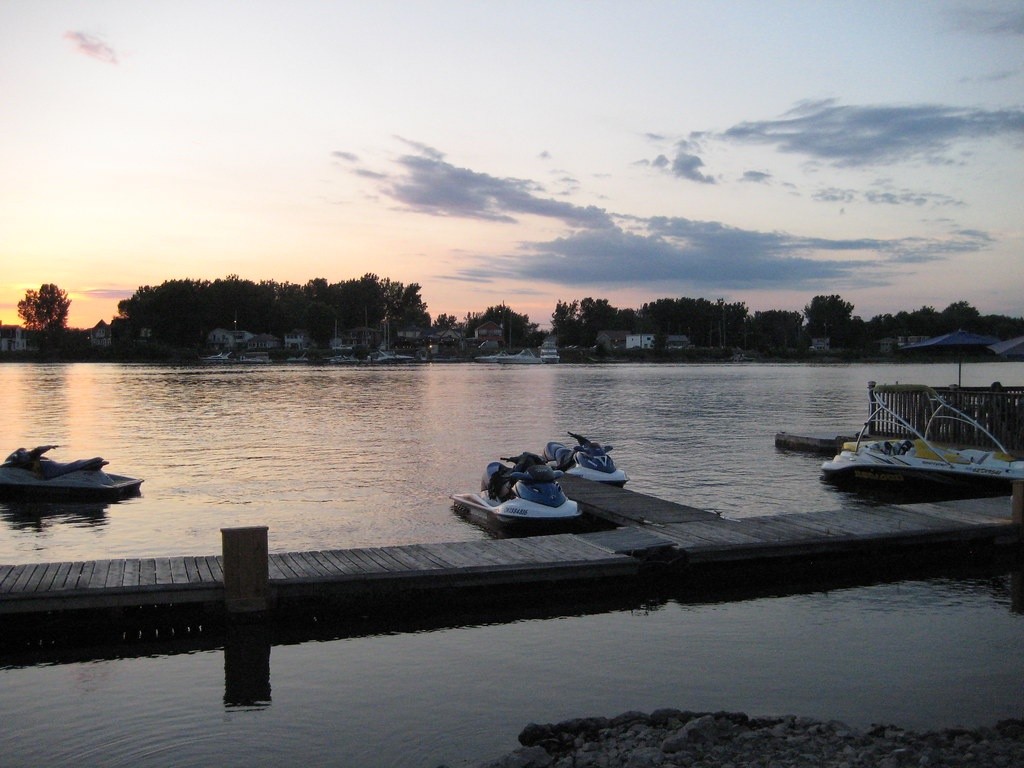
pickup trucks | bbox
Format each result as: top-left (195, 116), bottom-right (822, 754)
top-left (332, 345), bottom-right (352, 350)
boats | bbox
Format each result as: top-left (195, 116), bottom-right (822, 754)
top-left (366, 351), bottom-right (415, 364)
top-left (474, 351), bottom-right (510, 363)
top-left (324, 355), bottom-right (362, 366)
top-left (239, 355), bottom-right (272, 364)
top-left (200, 352), bottom-right (233, 364)
top-left (822, 384), bottom-right (1024, 503)
top-left (286, 352), bottom-right (308, 363)
top-left (497, 349), bottom-right (542, 363)
top-left (724, 353), bottom-right (754, 364)
top-left (539, 349), bottom-right (560, 363)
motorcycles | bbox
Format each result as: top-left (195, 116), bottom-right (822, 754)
top-left (538, 431), bottom-right (630, 488)
top-left (449, 452), bottom-right (583, 538)
top-left (1, 446), bottom-right (145, 502)
